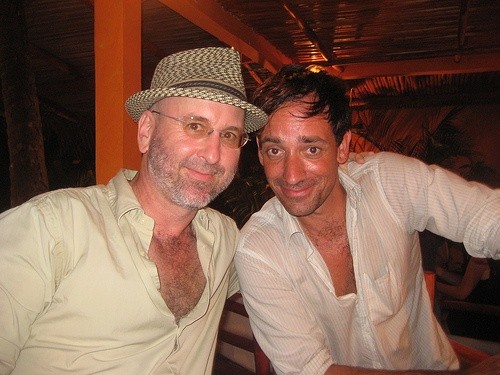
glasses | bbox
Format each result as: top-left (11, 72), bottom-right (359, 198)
top-left (151, 110), bottom-right (252, 149)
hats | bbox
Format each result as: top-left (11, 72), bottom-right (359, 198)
top-left (125, 47), bottom-right (269, 133)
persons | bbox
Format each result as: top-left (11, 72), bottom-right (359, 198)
top-left (0, 47), bottom-right (269, 375)
top-left (423, 103), bottom-right (500, 341)
top-left (234, 65), bottom-right (500, 375)
